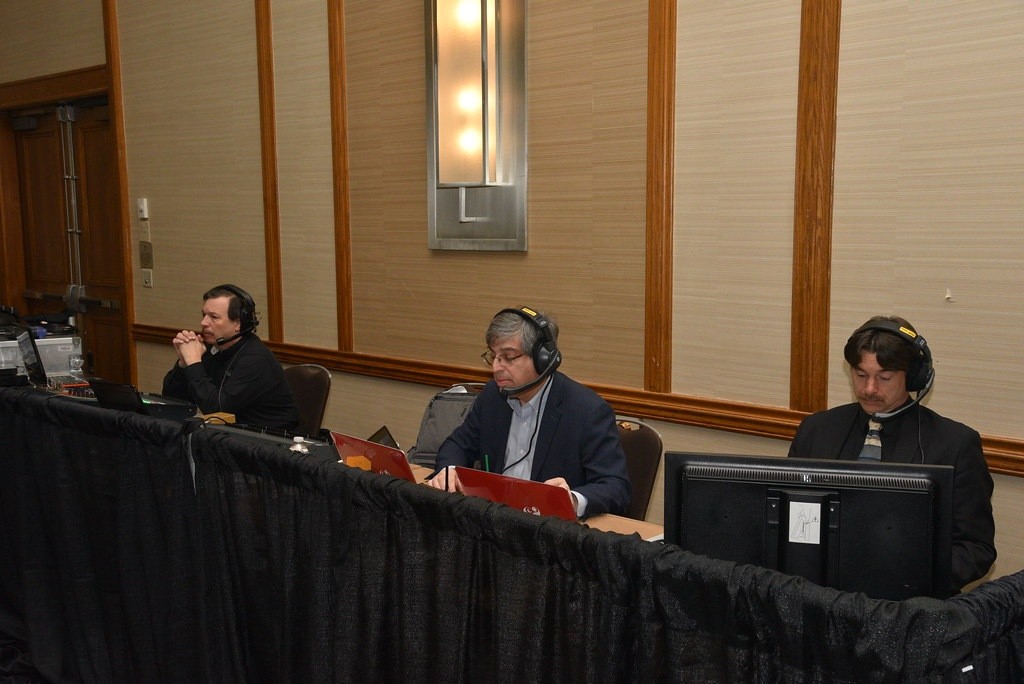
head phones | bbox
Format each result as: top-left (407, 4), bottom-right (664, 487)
top-left (215, 284), bottom-right (258, 332)
top-left (492, 305), bottom-right (562, 376)
top-left (848, 320), bottom-right (932, 393)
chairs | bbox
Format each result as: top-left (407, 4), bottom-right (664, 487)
top-left (616, 418), bottom-right (663, 517)
top-left (284, 364), bottom-right (332, 437)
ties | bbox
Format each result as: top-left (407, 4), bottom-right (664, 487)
top-left (859, 417), bottom-right (883, 462)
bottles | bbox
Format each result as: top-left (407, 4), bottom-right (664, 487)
top-left (287, 437), bottom-right (311, 454)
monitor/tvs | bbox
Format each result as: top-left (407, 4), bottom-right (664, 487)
top-left (662, 450), bottom-right (955, 602)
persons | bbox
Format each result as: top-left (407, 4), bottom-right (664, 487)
top-left (423, 306), bottom-right (631, 521)
top-left (786, 315), bottom-right (997, 597)
top-left (161, 284), bottom-right (304, 434)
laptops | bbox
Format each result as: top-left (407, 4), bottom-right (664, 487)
top-left (455, 465), bottom-right (578, 524)
top-left (329, 431), bottom-right (417, 485)
top-left (15, 327), bottom-right (89, 389)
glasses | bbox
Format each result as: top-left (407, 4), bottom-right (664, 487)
top-left (480, 345), bottom-right (529, 367)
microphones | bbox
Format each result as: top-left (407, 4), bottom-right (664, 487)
top-left (501, 351), bottom-right (562, 397)
top-left (216, 322), bottom-right (259, 347)
top-left (872, 367), bottom-right (936, 425)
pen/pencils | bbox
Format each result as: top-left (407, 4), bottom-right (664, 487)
top-left (445, 466), bottom-right (448, 491)
top-left (485, 454), bottom-right (489, 472)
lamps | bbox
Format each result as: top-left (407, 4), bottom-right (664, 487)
top-left (425, 0), bottom-right (529, 251)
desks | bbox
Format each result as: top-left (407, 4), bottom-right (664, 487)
top-left (410, 461), bottom-right (663, 541)
